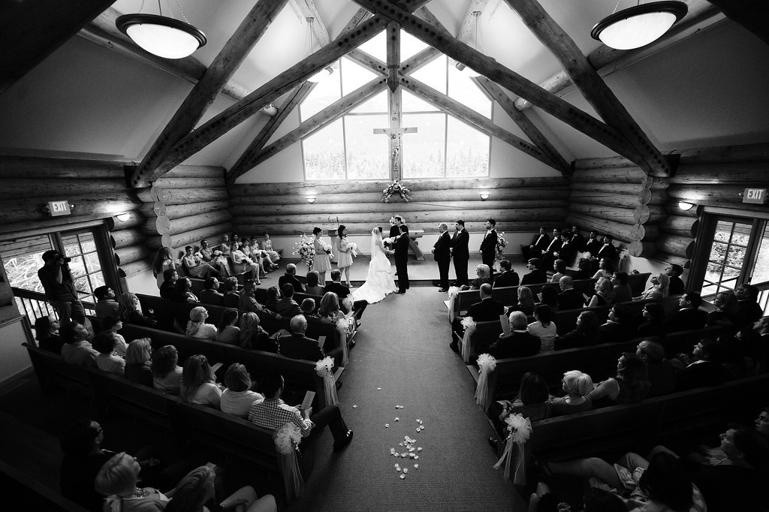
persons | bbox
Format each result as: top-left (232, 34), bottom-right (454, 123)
top-left (153, 233), bottom-right (281, 285)
top-left (35, 248), bottom-right (362, 455)
top-left (59, 414), bottom-right (280, 511)
top-left (350, 217), bottom-right (410, 304)
top-left (433, 219), bottom-right (768, 512)
top-left (311, 225), bottom-right (356, 288)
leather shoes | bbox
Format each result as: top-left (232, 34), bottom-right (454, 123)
top-left (433, 282), bottom-right (456, 292)
top-left (332, 430), bottom-right (353, 451)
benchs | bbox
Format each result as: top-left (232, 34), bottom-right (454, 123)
top-left (452, 273), bottom-right (768, 511)
top-left (520, 234), bottom-right (625, 271)
top-left (0, 277), bottom-right (363, 512)
top-left (182, 245), bottom-right (283, 279)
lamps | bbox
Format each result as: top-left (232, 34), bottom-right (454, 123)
top-left (115, 0), bottom-right (207, 60)
top-left (590, 0), bottom-right (689, 50)
